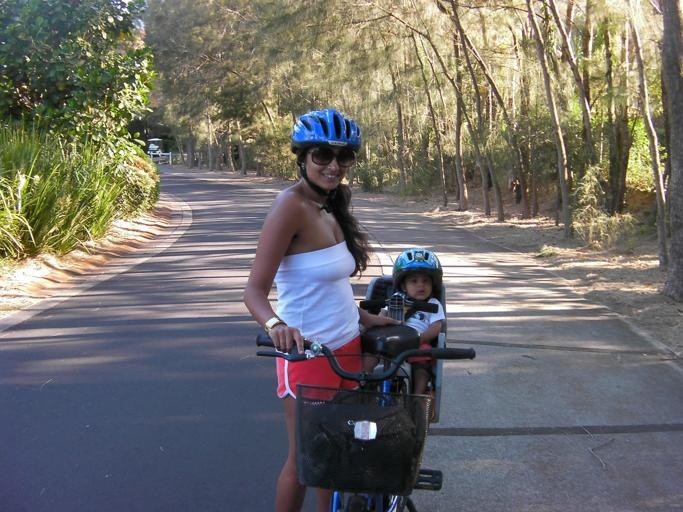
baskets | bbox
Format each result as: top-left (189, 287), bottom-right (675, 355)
top-left (296, 382), bottom-right (433, 496)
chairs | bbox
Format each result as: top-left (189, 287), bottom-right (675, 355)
top-left (363, 275), bottom-right (445, 423)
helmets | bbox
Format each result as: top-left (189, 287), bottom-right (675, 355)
top-left (290, 108), bottom-right (361, 154)
top-left (394, 249), bottom-right (442, 296)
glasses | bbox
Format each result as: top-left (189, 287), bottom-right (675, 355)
top-left (306, 147), bottom-right (355, 167)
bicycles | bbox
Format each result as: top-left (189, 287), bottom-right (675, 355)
top-left (254, 293), bottom-right (474, 510)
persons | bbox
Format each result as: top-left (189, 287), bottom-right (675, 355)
top-left (243, 107), bottom-right (402, 512)
top-left (364, 249), bottom-right (447, 394)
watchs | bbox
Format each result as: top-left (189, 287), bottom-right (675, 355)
top-left (262, 315), bottom-right (287, 335)
